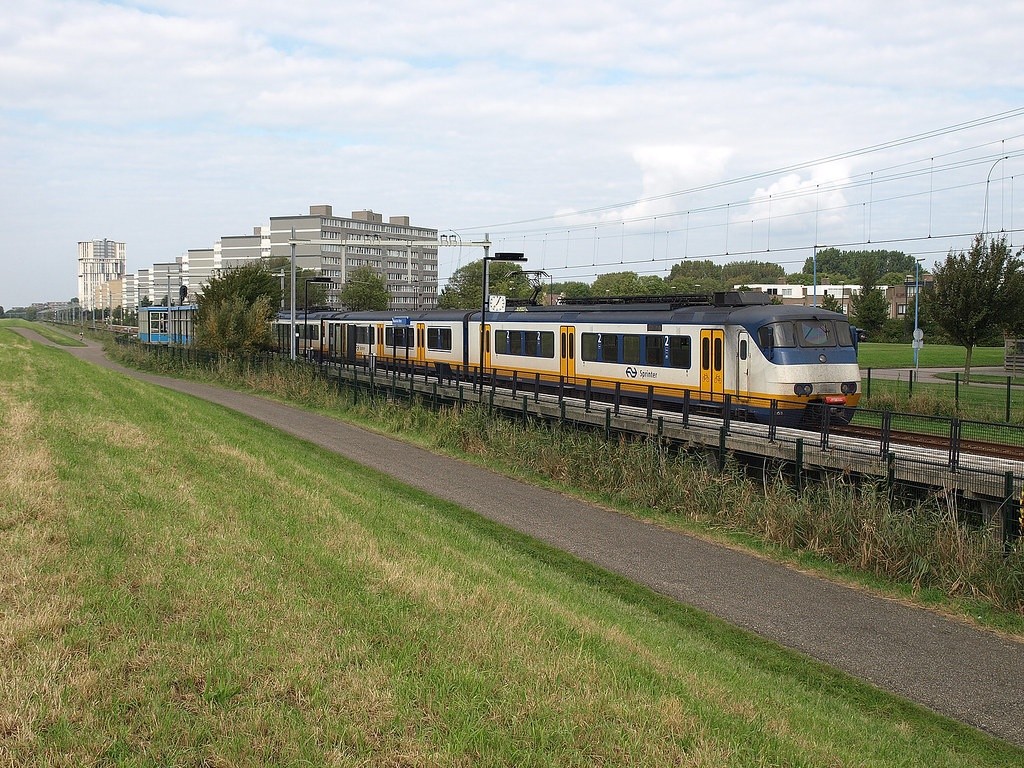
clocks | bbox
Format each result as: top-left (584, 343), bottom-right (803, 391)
top-left (488, 295), bottom-right (502, 313)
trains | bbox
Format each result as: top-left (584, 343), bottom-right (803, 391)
top-left (255, 290), bottom-right (864, 424)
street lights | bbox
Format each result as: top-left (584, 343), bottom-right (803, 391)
top-left (479, 253), bottom-right (528, 400)
top-left (914, 258), bottom-right (925, 382)
top-left (302, 277), bottom-right (334, 365)
